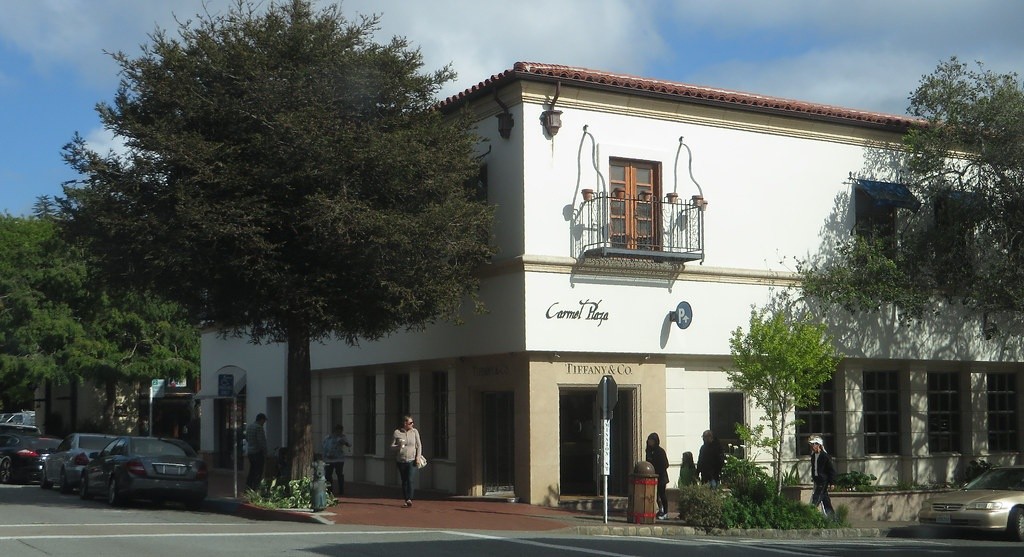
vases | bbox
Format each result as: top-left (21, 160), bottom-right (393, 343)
top-left (692, 195), bottom-right (703, 206)
top-left (640, 190), bottom-right (652, 201)
top-left (666, 192), bottom-right (678, 204)
top-left (614, 187), bottom-right (625, 199)
top-left (699, 200), bottom-right (709, 211)
top-left (582, 189), bottom-right (593, 201)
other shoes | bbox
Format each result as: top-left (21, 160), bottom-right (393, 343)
top-left (404, 502), bottom-right (407, 505)
top-left (407, 500), bottom-right (412, 506)
top-left (657, 513), bottom-right (669, 519)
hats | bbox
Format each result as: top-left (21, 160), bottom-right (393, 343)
top-left (255, 413), bottom-right (269, 420)
top-left (809, 436), bottom-right (823, 444)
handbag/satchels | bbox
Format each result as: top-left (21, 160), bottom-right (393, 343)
top-left (416, 455), bottom-right (428, 469)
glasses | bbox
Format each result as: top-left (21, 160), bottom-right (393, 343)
top-left (406, 420), bottom-right (414, 424)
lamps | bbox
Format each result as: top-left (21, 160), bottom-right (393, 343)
top-left (540, 81), bottom-right (564, 136)
top-left (493, 86), bottom-right (515, 140)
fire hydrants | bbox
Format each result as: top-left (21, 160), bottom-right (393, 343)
top-left (307, 459), bottom-right (332, 513)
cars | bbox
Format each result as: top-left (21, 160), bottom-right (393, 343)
top-left (40, 432), bottom-right (121, 494)
top-left (916, 465), bottom-right (1024, 543)
top-left (0, 410), bottom-right (64, 484)
top-left (78, 436), bottom-right (211, 506)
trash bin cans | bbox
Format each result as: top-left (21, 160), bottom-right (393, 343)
top-left (627, 461), bottom-right (659, 525)
top-left (325, 460), bottom-right (344, 496)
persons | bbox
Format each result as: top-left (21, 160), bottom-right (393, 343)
top-left (390, 416), bottom-right (422, 508)
top-left (808, 435), bottom-right (839, 515)
top-left (244, 413), bottom-right (267, 491)
top-left (646, 430), bottom-right (725, 520)
top-left (322, 424), bottom-right (352, 495)
